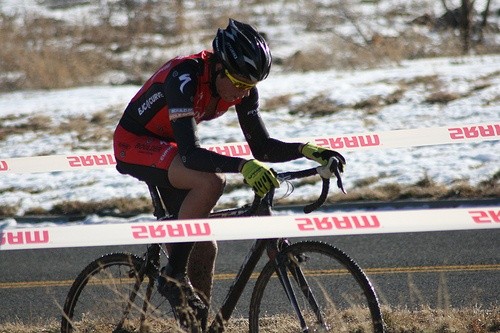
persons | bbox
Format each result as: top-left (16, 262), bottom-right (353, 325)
top-left (112, 18), bottom-right (347, 332)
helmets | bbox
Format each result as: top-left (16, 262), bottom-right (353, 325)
top-left (212, 17), bottom-right (272, 83)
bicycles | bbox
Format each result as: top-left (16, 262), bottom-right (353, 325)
top-left (62, 156), bottom-right (384, 333)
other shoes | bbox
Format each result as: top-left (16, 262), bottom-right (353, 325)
top-left (157, 265), bottom-right (208, 311)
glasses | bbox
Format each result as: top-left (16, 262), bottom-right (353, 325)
top-left (224, 69), bottom-right (256, 91)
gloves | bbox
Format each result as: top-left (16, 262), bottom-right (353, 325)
top-left (301, 142), bottom-right (346, 177)
top-left (240, 159), bottom-right (281, 198)
